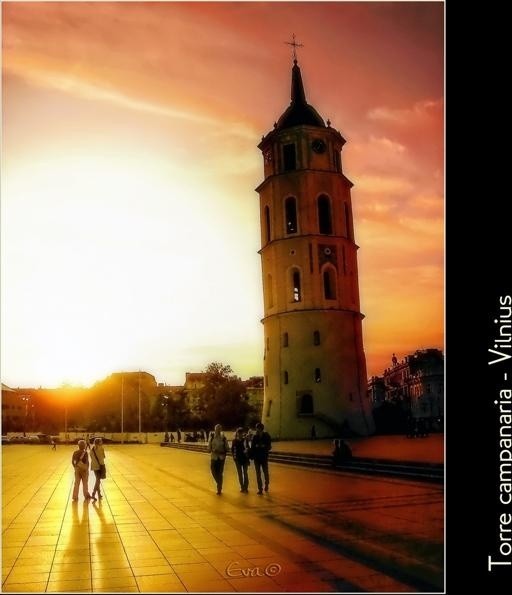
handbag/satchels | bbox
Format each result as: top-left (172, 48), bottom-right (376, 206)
top-left (99, 464), bottom-right (106, 479)
top-left (77, 461), bottom-right (88, 470)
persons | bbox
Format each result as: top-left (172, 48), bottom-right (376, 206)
top-left (23, 432), bottom-right (26, 438)
top-left (89, 438), bottom-right (105, 501)
top-left (71, 440), bottom-right (92, 500)
top-left (52, 439), bottom-right (57, 451)
top-left (405, 416), bottom-right (429, 438)
top-left (231, 427), bottom-right (251, 493)
top-left (250, 423), bottom-right (271, 495)
top-left (246, 430), bottom-right (254, 441)
top-left (164, 429), bottom-right (215, 443)
top-left (338, 439), bottom-right (352, 459)
top-left (207, 424), bottom-right (229, 495)
top-left (332, 439), bottom-right (341, 470)
top-left (85, 434), bottom-right (91, 451)
